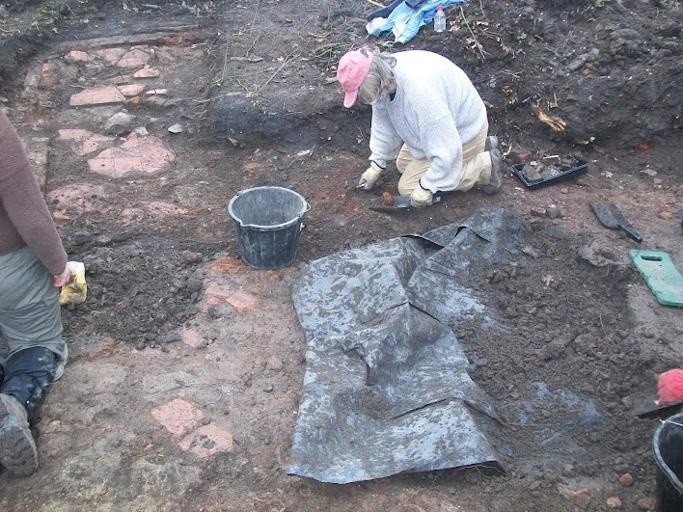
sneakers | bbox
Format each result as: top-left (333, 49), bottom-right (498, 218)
top-left (484, 149), bottom-right (503, 194)
top-left (0, 391), bottom-right (38, 477)
top-left (485, 135), bottom-right (499, 151)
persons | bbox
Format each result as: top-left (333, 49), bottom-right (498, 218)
top-left (335, 47), bottom-right (503, 211)
top-left (0, 105), bottom-right (71, 479)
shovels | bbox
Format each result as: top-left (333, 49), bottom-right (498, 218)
top-left (590, 200), bottom-right (642, 243)
top-left (372, 192), bottom-right (410, 215)
top-left (619, 365), bottom-right (683, 418)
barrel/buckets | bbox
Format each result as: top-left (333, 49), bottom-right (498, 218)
top-left (653, 409), bottom-right (682, 512)
top-left (228, 187), bottom-right (310, 272)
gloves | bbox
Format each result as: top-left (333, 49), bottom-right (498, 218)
top-left (409, 180), bottom-right (434, 209)
top-left (359, 160), bottom-right (386, 192)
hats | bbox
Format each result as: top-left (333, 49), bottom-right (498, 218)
top-left (337, 48), bottom-right (372, 108)
